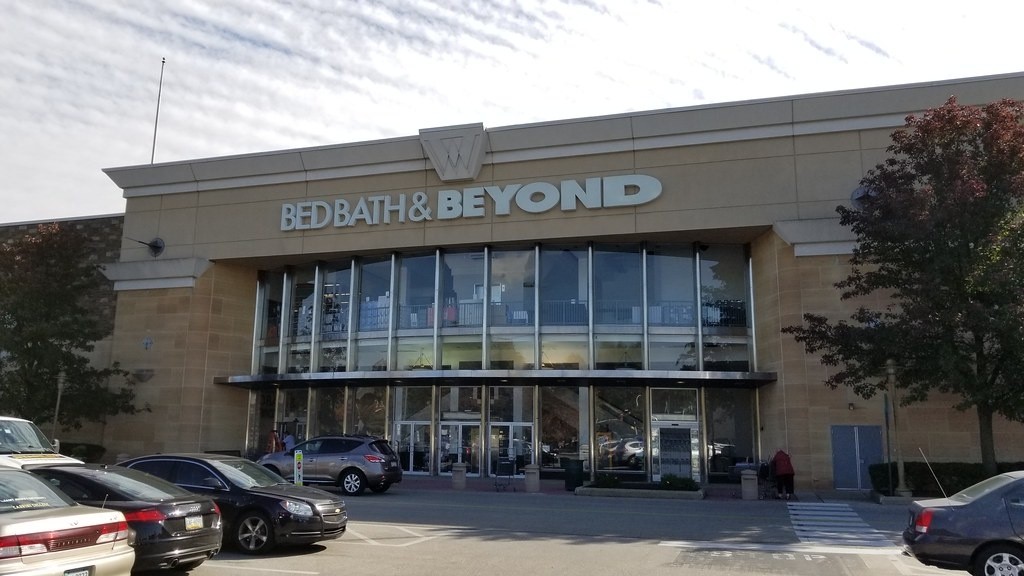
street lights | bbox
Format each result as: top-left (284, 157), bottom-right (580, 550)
top-left (878, 359), bottom-right (913, 498)
top-left (50, 370), bottom-right (72, 444)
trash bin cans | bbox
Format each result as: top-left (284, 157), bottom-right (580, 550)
top-left (451, 463), bottom-right (466, 489)
top-left (525, 464), bottom-right (541, 493)
top-left (740, 469), bottom-right (759, 501)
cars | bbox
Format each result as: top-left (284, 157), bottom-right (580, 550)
top-left (113, 454), bottom-right (347, 556)
top-left (398, 433), bottom-right (558, 471)
top-left (599, 438), bottom-right (735, 473)
top-left (902, 471), bottom-right (1024, 576)
top-left (0, 464), bottom-right (223, 576)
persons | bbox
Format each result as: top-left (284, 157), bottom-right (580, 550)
top-left (280, 431), bottom-right (296, 453)
top-left (772, 449), bottom-right (795, 500)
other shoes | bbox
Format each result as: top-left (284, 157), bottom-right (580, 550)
top-left (778, 496), bottom-right (783, 499)
top-left (786, 496), bottom-right (790, 500)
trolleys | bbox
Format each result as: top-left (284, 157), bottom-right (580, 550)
top-left (731, 454), bottom-right (773, 500)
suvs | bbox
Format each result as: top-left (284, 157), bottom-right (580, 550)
top-left (256, 434), bottom-right (400, 496)
top-left (0, 416), bottom-right (86, 470)
